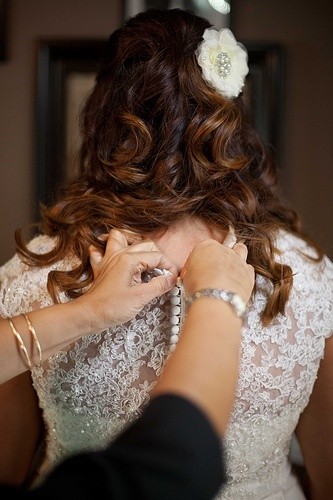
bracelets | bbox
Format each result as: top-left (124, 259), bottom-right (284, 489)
top-left (20, 313), bottom-right (44, 368)
top-left (7, 318), bottom-right (32, 372)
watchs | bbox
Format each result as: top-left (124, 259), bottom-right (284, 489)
top-left (184, 287), bottom-right (248, 327)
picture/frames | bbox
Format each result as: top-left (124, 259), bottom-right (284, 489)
top-left (30, 32), bottom-right (290, 228)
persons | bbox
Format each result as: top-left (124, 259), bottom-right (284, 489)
top-left (0, 229), bottom-right (257, 499)
top-left (0, 6), bottom-right (333, 500)
